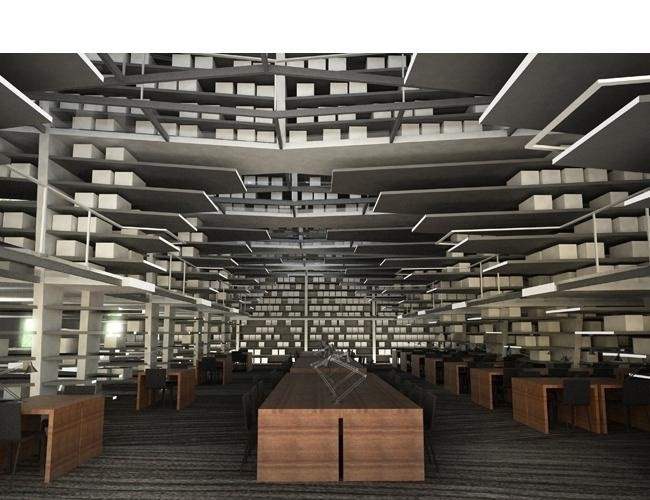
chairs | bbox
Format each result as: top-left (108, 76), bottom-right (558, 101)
top-left (241, 350), bottom-right (437, 482)
top-left (137, 347), bottom-right (248, 410)
top-left (1, 385), bottom-right (104, 484)
top-left (392, 346), bottom-right (648, 435)
